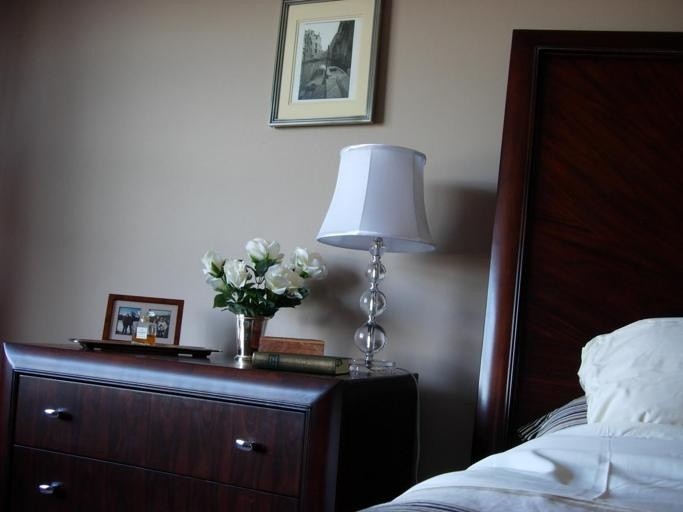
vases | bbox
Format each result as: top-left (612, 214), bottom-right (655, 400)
top-left (233, 313), bottom-right (268, 369)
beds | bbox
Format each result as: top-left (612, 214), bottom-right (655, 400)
top-left (365, 30), bottom-right (683, 512)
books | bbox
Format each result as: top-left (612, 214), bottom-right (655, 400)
top-left (250, 350), bottom-right (353, 376)
top-left (258, 337), bottom-right (325, 356)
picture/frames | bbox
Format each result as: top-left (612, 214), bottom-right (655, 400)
top-left (268, 0), bottom-right (384, 127)
top-left (102, 293), bottom-right (185, 344)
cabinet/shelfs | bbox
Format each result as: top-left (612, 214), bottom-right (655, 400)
top-left (1, 340), bottom-right (419, 512)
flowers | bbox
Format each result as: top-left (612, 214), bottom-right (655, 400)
top-left (199, 236), bottom-right (329, 355)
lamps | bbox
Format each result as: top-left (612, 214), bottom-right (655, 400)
top-left (315, 142), bottom-right (436, 375)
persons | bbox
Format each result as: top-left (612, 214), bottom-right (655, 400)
top-left (149, 315), bottom-right (168, 338)
top-left (122, 313), bottom-right (135, 335)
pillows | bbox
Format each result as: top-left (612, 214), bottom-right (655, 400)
top-left (516, 316), bottom-right (681, 444)
